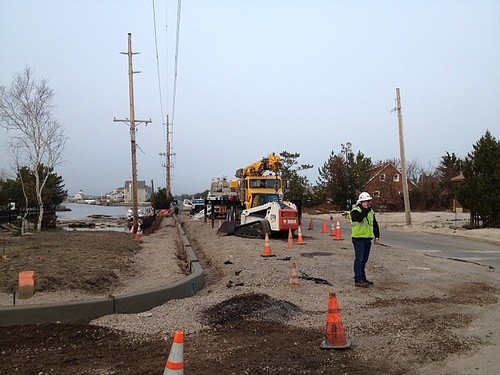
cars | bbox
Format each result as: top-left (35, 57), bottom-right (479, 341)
top-left (182, 197), bottom-right (205, 215)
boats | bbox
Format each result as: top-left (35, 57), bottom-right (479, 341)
top-left (125, 206), bottom-right (144, 219)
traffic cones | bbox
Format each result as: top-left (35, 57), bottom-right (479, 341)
top-left (129, 222), bottom-right (135, 234)
top-left (261, 232), bottom-right (276, 256)
top-left (307, 218), bottom-right (315, 229)
top-left (321, 218), bottom-right (327, 233)
top-left (333, 220), bottom-right (345, 240)
top-left (296, 224), bottom-right (306, 245)
top-left (288, 261), bottom-right (301, 287)
top-left (287, 227), bottom-right (295, 249)
top-left (329, 220), bottom-right (336, 235)
top-left (136, 223), bottom-right (141, 241)
top-left (314, 291), bottom-right (354, 349)
top-left (161, 329), bottom-right (184, 375)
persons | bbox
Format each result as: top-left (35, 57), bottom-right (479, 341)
top-left (349, 192), bottom-right (380, 287)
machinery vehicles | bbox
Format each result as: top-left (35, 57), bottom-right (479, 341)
top-left (203, 152), bottom-right (299, 240)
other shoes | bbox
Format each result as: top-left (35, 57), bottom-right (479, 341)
top-left (355, 280), bottom-right (369, 286)
top-left (363, 279), bottom-right (373, 285)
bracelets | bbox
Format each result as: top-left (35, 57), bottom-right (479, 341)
top-left (375, 238), bottom-right (379, 239)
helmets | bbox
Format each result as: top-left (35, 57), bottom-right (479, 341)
top-left (359, 192), bottom-right (372, 202)
